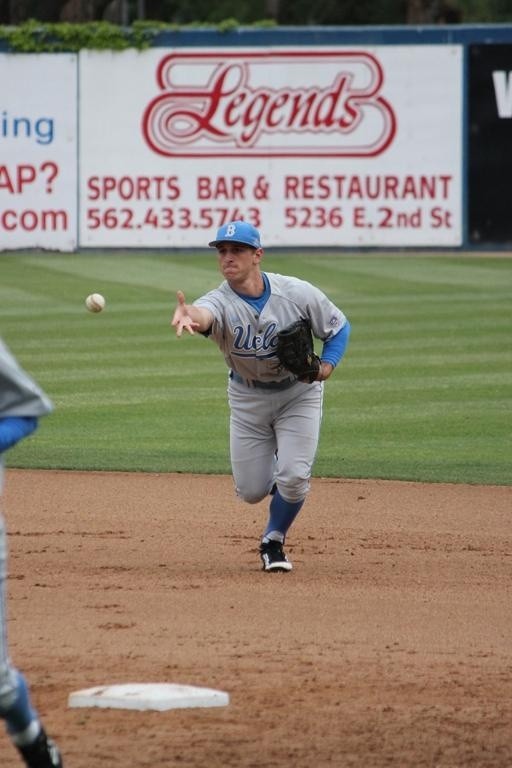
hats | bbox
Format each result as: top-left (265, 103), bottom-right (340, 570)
top-left (208, 217), bottom-right (263, 252)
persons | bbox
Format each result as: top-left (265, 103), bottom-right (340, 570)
top-left (170, 219), bottom-right (351, 573)
top-left (0, 337), bottom-right (64, 767)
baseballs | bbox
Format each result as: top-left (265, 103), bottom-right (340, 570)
top-left (84, 293), bottom-right (105, 312)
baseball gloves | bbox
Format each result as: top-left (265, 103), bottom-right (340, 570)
top-left (277, 320), bottom-right (321, 382)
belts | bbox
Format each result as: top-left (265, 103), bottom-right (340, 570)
top-left (228, 366), bottom-right (302, 392)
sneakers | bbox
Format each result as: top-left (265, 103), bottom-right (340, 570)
top-left (21, 741), bottom-right (65, 768)
top-left (259, 529), bottom-right (297, 575)
top-left (268, 480), bottom-right (279, 496)
